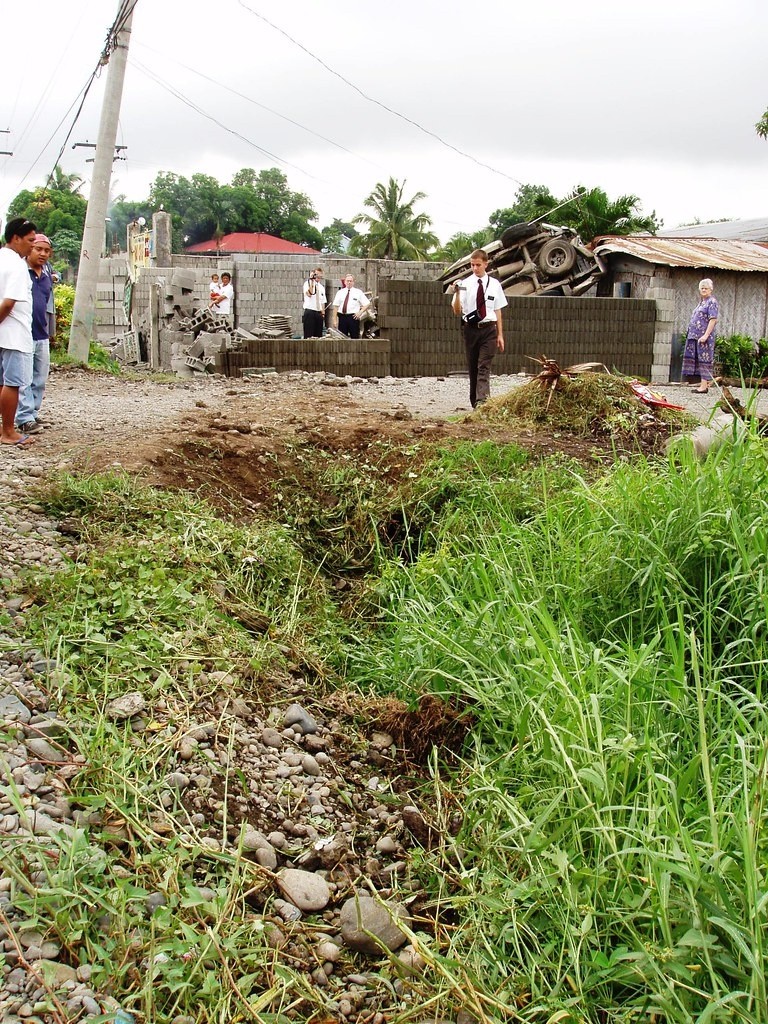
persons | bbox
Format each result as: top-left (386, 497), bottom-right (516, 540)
top-left (208, 274), bottom-right (221, 308)
top-left (209, 272), bottom-right (233, 319)
top-left (0, 217), bottom-right (36, 445)
top-left (451, 249), bottom-right (508, 410)
top-left (44, 260), bottom-right (61, 340)
top-left (333, 273), bottom-right (370, 340)
top-left (302, 268), bottom-right (327, 340)
top-left (13, 234), bottom-right (53, 434)
top-left (680, 278), bottom-right (719, 393)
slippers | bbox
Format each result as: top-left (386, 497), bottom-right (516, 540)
top-left (2, 435), bottom-right (36, 445)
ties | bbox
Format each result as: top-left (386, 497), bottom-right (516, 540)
top-left (316, 284), bottom-right (320, 310)
top-left (342, 290), bottom-right (350, 314)
top-left (476, 279), bottom-right (486, 319)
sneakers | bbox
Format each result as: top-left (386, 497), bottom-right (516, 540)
top-left (35, 417), bottom-right (51, 427)
top-left (17, 421), bottom-right (43, 434)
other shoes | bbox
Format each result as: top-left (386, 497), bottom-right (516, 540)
top-left (476, 401), bottom-right (485, 409)
top-left (691, 388), bottom-right (708, 393)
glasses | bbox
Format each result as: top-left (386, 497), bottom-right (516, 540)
top-left (16, 220), bottom-right (30, 231)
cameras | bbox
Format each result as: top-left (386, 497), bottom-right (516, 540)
top-left (455, 283), bottom-right (466, 291)
top-left (312, 274), bottom-right (317, 279)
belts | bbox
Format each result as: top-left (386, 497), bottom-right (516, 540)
top-left (470, 321), bottom-right (495, 328)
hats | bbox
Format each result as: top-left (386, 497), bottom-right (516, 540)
top-left (33, 234), bottom-right (51, 246)
